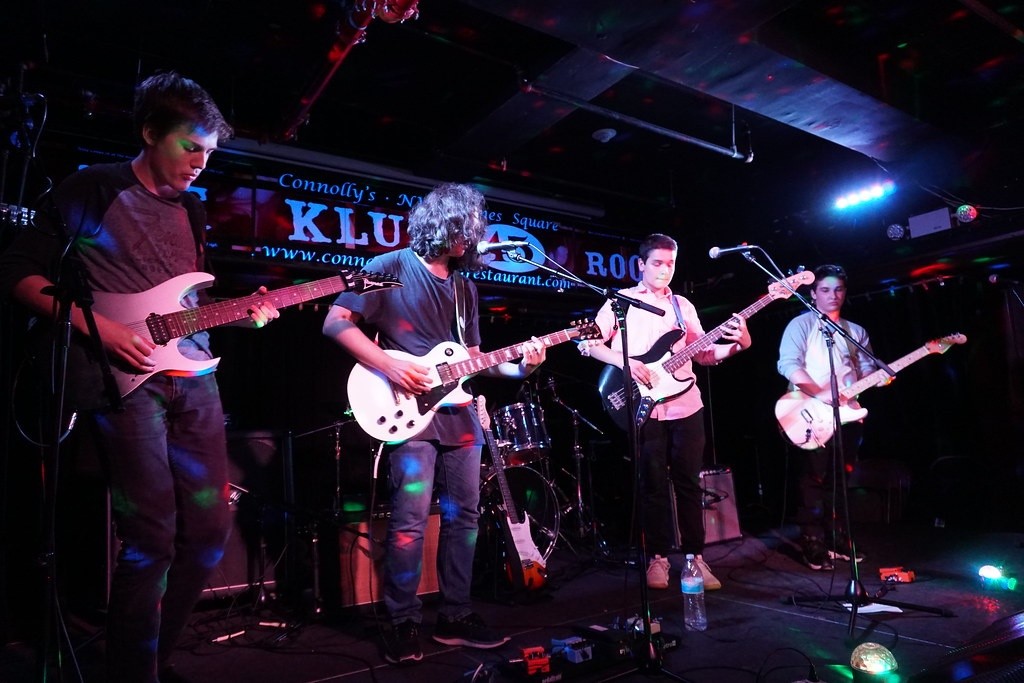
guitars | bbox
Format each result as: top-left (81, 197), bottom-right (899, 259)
top-left (89, 267), bottom-right (408, 401)
top-left (348, 318), bottom-right (600, 445)
top-left (599, 271), bottom-right (815, 434)
top-left (473, 393), bottom-right (547, 590)
top-left (773, 334), bottom-right (969, 448)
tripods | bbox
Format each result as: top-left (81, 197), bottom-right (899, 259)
top-left (507, 250), bottom-right (684, 682)
top-left (739, 252), bottom-right (952, 649)
top-left (272, 416), bottom-right (358, 642)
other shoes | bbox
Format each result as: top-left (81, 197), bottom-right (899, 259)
top-left (157, 665), bottom-right (192, 683)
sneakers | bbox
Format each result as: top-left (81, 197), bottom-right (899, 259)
top-left (432, 612), bottom-right (512, 649)
top-left (645, 554), bottom-right (670, 589)
top-left (825, 546), bottom-right (865, 563)
top-left (384, 618), bottom-right (423, 664)
top-left (802, 545), bottom-right (834, 570)
top-left (680, 553), bottom-right (721, 590)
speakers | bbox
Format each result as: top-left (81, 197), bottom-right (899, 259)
top-left (667, 465), bottom-right (742, 551)
top-left (103, 428), bottom-right (289, 609)
top-left (315, 500), bottom-right (447, 625)
top-left (908, 609), bottom-right (1024, 683)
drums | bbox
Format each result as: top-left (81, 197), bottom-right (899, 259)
top-left (471, 401), bottom-right (586, 571)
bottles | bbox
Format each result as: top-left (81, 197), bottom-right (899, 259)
top-left (681, 554), bottom-right (708, 632)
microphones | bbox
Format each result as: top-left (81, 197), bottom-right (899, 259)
top-left (477, 240), bottom-right (531, 256)
top-left (989, 275), bottom-right (1019, 284)
top-left (516, 375), bottom-right (532, 402)
top-left (700, 495), bottom-right (729, 508)
top-left (708, 245), bottom-right (758, 258)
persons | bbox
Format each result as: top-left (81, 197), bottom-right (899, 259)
top-left (586, 233), bottom-right (751, 590)
top-left (777, 264), bottom-right (896, 569)
top-left (321, 182), bottom-right (544, 661)
top-left (12, 71), bottom-right (281, 683)
